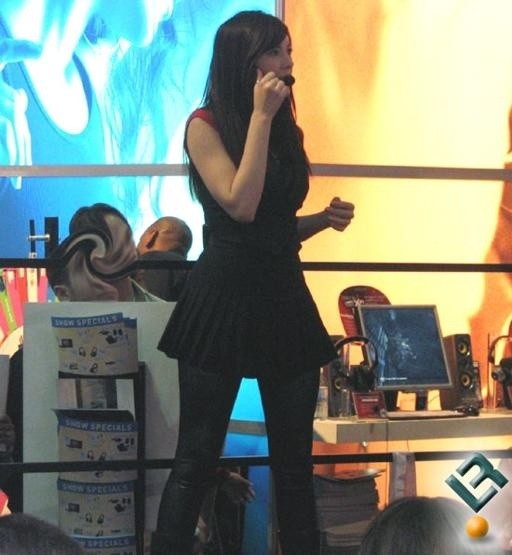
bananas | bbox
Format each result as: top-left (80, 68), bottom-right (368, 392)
top-left (386, 410), bottom-right (466, 420)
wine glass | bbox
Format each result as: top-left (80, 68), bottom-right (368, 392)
top-left (254, 79), bottom-right (260, 84)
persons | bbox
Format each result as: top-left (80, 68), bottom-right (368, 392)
top-left (1, 346), bottom-right (25, 513)
top-left (134, 214), bottom-right (191, 301)
top-left (208, 463), bottom-right (257, 507)
top-left (150, 9), bottom-right (356, 555)
top-left (356, 495), bottom-right (487, 554)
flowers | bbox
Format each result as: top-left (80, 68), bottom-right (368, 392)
top-left (280, 74), bottom-right (296, 86)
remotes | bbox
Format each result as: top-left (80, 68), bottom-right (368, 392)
top-left (453, 406), bottom-right (479, 417)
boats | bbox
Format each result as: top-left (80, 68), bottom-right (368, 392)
top-left (439, 333), bottom-right (483, 411)
top-left (318, 335), bottom-right (352, 417)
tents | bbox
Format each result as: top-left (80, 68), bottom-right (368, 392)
top-left (489, 335), bottom-right (512, 383)
top-left (334, 336), bottom-right (378, 394)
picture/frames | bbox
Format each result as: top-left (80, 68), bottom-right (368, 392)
top-left (225, 405), bottom-right (512, 555)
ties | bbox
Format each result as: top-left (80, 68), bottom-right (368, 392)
top-left (355, 303), bottom-right (453, 411)
